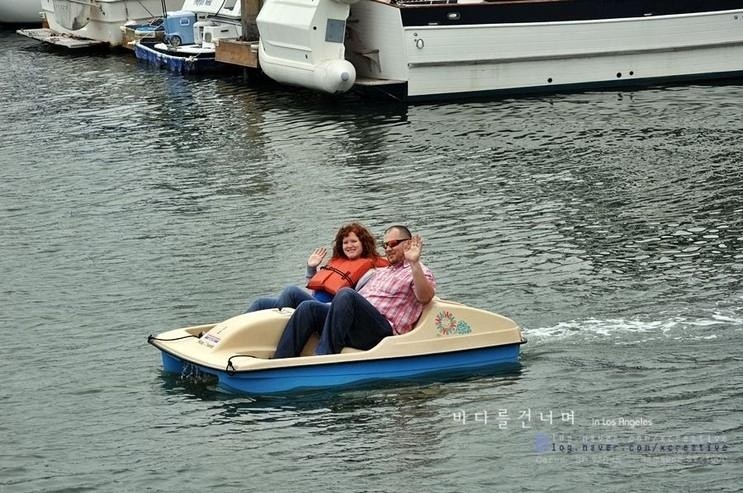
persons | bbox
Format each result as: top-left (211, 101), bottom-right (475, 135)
top-left (199, 223), bottom-right (389, 339)
top-left (274, 226), bottom-right (436, 358)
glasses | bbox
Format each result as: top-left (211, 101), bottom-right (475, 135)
top-left (382, 238), bottom-right (408, 249)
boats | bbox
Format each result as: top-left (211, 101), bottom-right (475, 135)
top-left (147, 296), bottom-right (527, 397)
